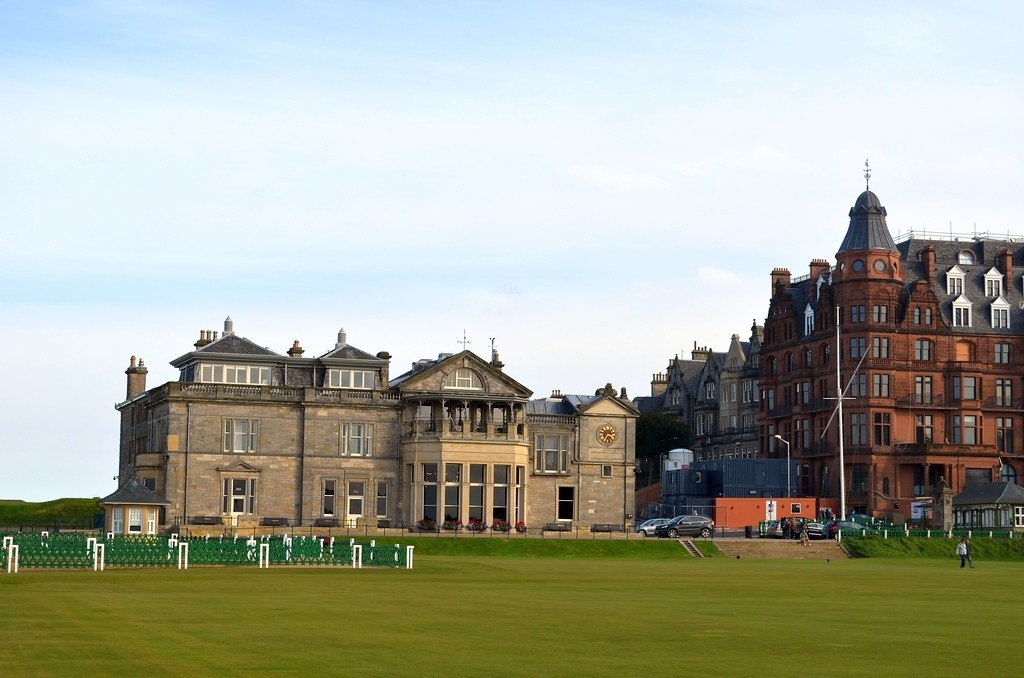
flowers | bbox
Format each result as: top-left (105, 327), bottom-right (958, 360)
top-left (467, 519), bottom-right (488, 530)
top-left (491, 519), bottom-right (512, 532)
top-left (442, 520), bottom-right (463, 529)
top-left (515, 520), bottom-right (527, 532)
top-left (416, 519), bottom-right (437, 529)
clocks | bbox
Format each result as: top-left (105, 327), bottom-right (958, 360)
top-left (596, 423), bottom-right (618, 447)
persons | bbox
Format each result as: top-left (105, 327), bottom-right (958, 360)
top-left (780, 507), bottom-right (842, 546)
top-left (964, 540), bottom-right (975, 569)
top-left (957, 539), bottom-right (966, 568)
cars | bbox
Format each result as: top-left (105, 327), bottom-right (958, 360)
top-left (766, 516), bottom-right (878, 540)
top-left (635, 518), bottom-right (671, 536)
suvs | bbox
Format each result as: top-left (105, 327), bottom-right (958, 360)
top-left (655, 514), bottom-right (714, 539)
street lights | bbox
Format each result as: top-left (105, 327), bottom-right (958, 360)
top-left (775, 434), bottom-right (792, 497)
